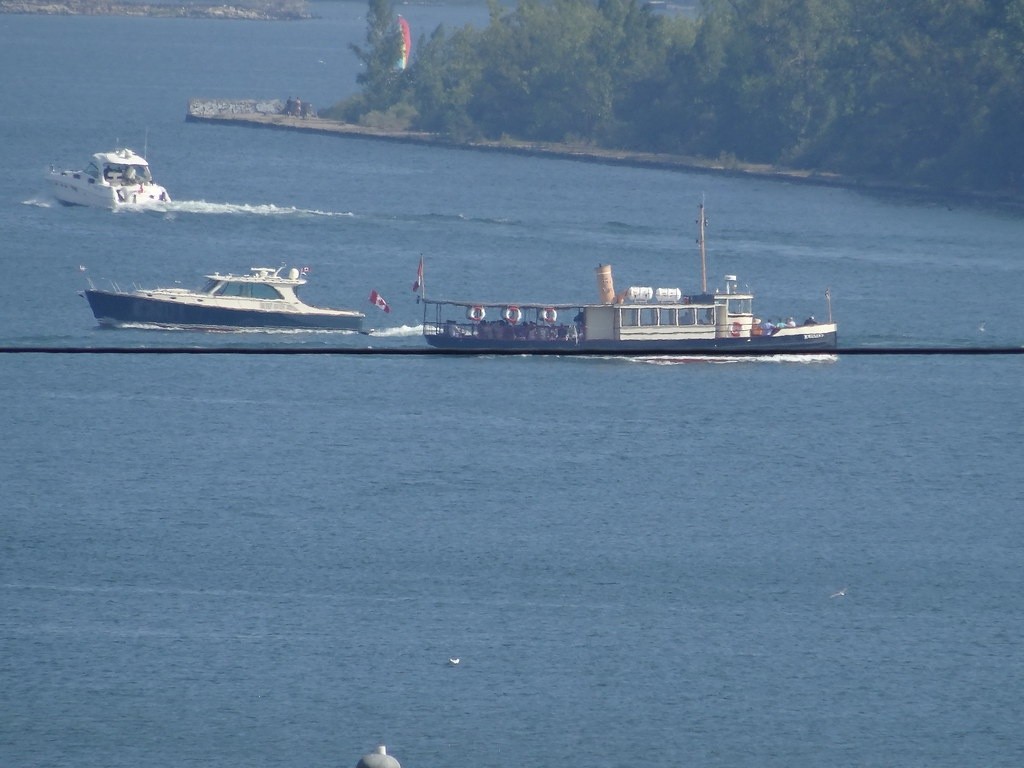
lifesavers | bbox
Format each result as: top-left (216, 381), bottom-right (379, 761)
top-left (465, 306), bottom-right (485, 321)
top-left (537, 308), bottom-right (558, 323)
top-left (499, 306), bottom-right (522, 322)
top-left (730, 321), bottom-right (743, 336)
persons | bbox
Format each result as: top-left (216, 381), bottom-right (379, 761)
top-left (763, 315), bottom-right (817, 336)
top-left (104, 165), bottom-right (136, 186)
top-left (444, 320), bottom-right (569, 339)
top-left (681, 311), bottom-right (692, 324)
top-left (286, 95), bottom-right (302, 118)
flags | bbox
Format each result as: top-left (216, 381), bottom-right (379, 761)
top-left (302, 266), bottom-right (312, 273)
top-left (368, 288), bottom-right (392, 315)
top-left (412, 256), bottom-right (425, 292)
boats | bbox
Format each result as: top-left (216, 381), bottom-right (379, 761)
top-left (81, 266), bottom-right (374, 335)
top-left (417, 203), bottom-right (841, 353)
top-left (52, 147), bottom-right (172, 214)
